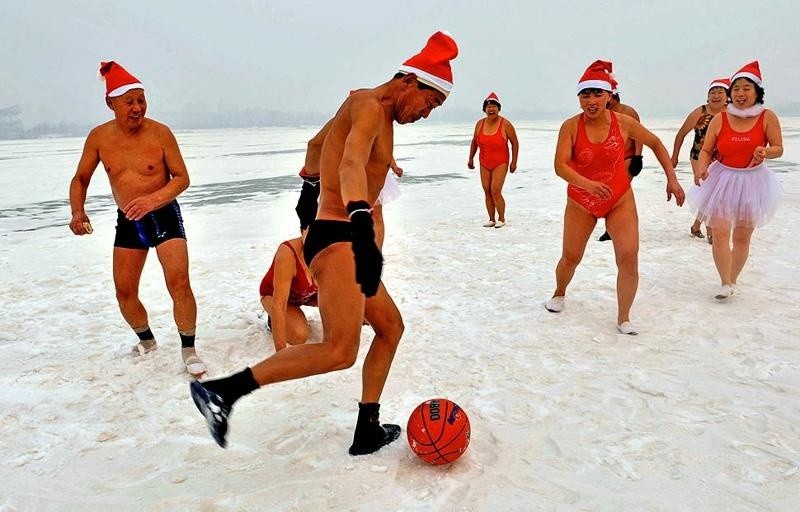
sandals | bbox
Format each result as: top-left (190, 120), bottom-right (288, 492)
top-left (348, 423), bottom-right (402, 456)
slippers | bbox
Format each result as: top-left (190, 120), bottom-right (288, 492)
top-left (189, 378), bottom-right (233, 450)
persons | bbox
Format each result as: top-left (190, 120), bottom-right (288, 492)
top-left (467, 92), bottom-right (518, 228)
top-left (598, 79), bottom-right (643, 242)
top-left (669, 78), bottom-right (732, 242)
top-left (68, 60), bottom-right (208, 377)
top-left (546, 60), bottom-right (686, 336)
top-left (192, 32), bottom-right (459, 453)
top-left (686, 61), bottom-right (784, 302)
top-left (260, 221), bottom-right (370, 352)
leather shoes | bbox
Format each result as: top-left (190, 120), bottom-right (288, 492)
top-left (691, 226), bottom-right (705, 238)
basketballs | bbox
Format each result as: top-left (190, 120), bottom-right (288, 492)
top-left (406, 394), bottom-right (473, 464)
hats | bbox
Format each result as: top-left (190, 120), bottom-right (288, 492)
top-left (576, 59), bottom-right (614, 96)
top-left (95, 59), bottom-right (145, 98)
top-left (398, 29), bottom-right (459, 92)
top-left (731, 60), bottom-right (763, 86)
top-left (707, 77), bottom-right (730, 92)
top-left (611, 78), bottom-right (621, 95)
top-left (484, 91), bottom-right (500, 104)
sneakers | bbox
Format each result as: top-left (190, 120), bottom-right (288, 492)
top-left (184, 351), bottom-right (208, 377)
top-left (483, 220), bottom-right (496, 227)
top-left (714, 284), bottom-right (733, 300)
top-left (127, 339), bottom-right (157, 359)
top-left (495, 220), bottom-right (506, 228)
top-left (545, 293), bottom-right (567, 313)
top-left (616, 320), bottom-right (639, 335)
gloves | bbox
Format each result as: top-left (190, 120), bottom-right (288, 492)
top-left (628, 155), bottom-right (643, 177)
top-left (346, 200), bottom-right (384, 299)
